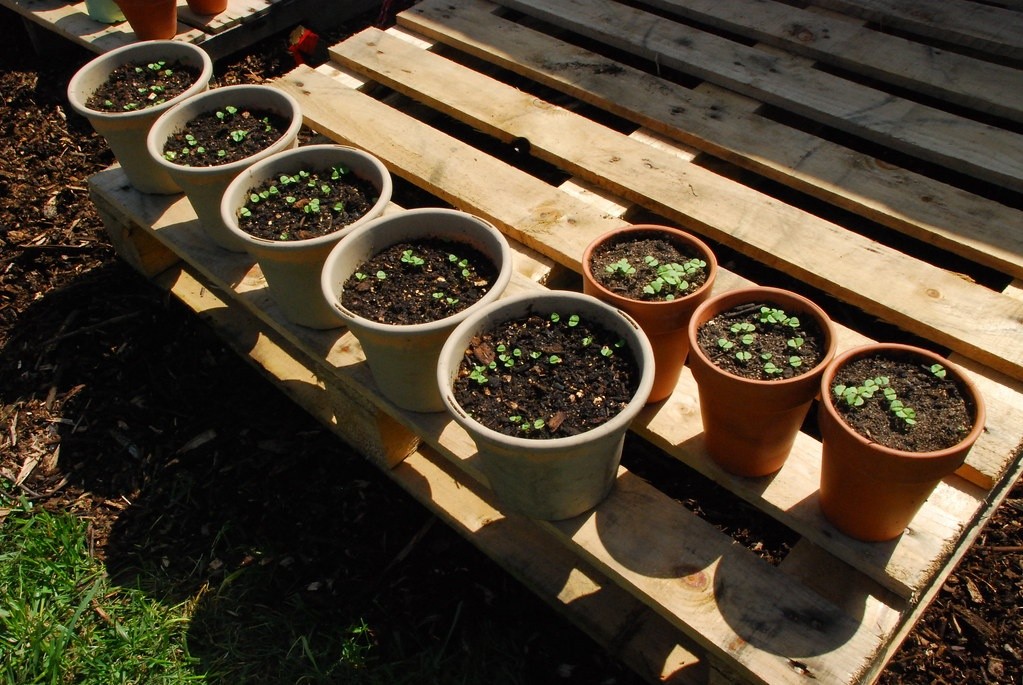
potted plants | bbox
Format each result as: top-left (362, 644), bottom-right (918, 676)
top-left (67, 40), bottom-right (213, 194)
top-left (436, 288), bottom-right (657, 522)
top-left (816, 342), bottom-right (986, 543)
top-left (320, 208), bottom-right (512, 415)
top-left (145, 84), bottom-right (303, 251)
top-left (219, 144), bottom-right (393, 332)
top-left (582, 224), bottom-right (716, 405)
top-left (687, 285), bottom-right (836, 479)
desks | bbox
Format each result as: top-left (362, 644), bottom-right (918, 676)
top-left (90, 0), bottom-right (1022, 685)
top-left (0, 0), bottom-right (309, 62)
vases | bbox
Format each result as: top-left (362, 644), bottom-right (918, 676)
top-left (14, 0), bottom-right (228, 40)
